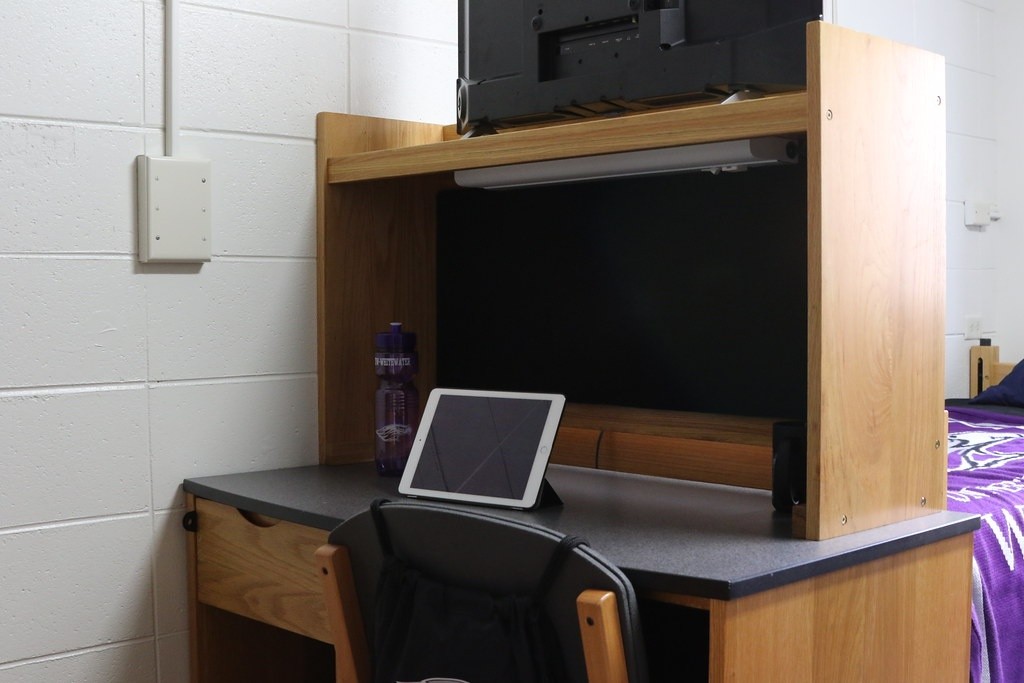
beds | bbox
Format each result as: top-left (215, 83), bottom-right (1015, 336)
top-left (946, 345), bottom-right (1024, 683)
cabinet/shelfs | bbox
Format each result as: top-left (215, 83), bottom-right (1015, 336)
top-left (311, 20), bottom-right (951, 539)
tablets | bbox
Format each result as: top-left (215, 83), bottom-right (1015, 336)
top-left (396, 389), bottom-right (566, 509)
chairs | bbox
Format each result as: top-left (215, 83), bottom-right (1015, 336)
top-left (312, 497), bottom-right (647, 683)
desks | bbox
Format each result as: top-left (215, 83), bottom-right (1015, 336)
top-left (178, 464), bottom-right (982, 683)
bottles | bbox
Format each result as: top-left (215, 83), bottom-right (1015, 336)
top-left (374, 322), bottom-right (419, 476)
